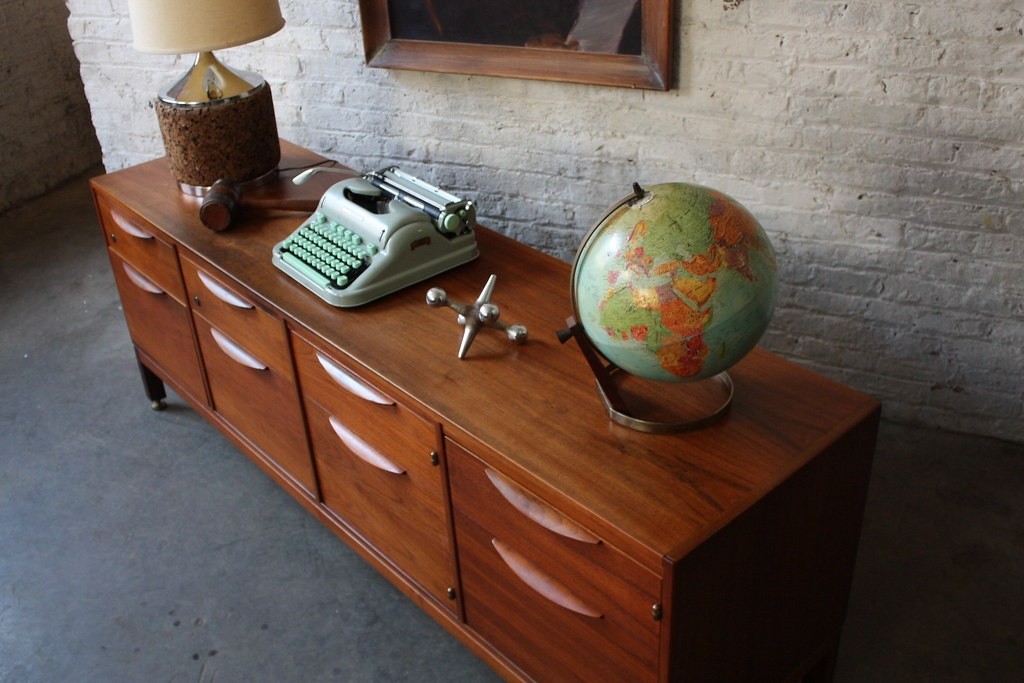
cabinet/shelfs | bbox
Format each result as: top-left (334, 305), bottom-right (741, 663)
top-left (89, 137), bottom-right (879, 683)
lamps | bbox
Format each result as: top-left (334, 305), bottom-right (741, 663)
top-left (127, 0), bottom-right (287, 198)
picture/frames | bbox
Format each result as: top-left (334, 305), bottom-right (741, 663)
top-left (359, 0), bottom-right (676, 91)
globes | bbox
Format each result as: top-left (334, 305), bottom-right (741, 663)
top-left (560, 182), bottom-right (778, 434)
